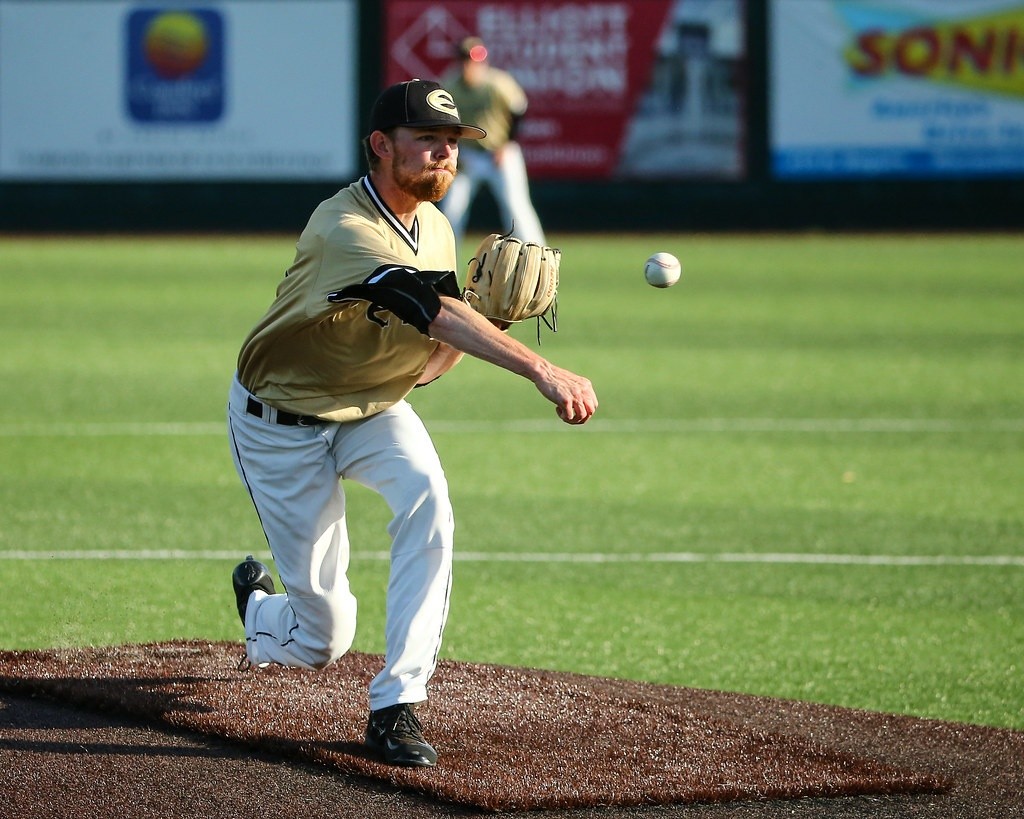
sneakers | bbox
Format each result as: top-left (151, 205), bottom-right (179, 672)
top-left (232, 557), bottom-right (275, 672)
top-left (363, 703), bottom-right (438, 766)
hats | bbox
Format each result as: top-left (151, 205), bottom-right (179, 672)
top-left (458, 37), bottom-right (486, 59)
top-left (369, 79), bottom-right (486, 140)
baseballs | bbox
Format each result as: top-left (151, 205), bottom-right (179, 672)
top-left (645, 251), bottom-right (682, 290)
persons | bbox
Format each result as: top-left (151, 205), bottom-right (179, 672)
top-left (228, 78), bottom-right (598, 766)
top-left (441, 36), bottom-right (545, 248)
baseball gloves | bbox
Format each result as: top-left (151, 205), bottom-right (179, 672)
top-left (462, 235), bottom-right (561, 334)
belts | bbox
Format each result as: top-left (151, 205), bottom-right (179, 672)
top-left (247, 397), bottom-right (330, 427)
